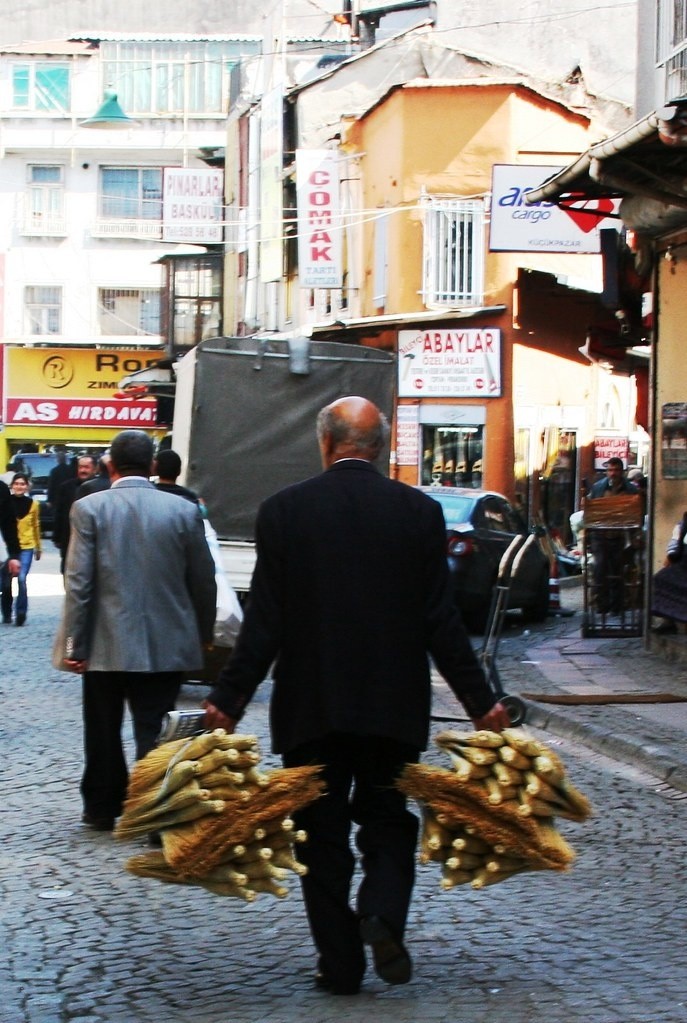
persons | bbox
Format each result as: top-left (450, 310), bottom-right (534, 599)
top-left (570, 457), bottom-right (648, 617)
top-left (46, 450), bottom-right (200, 584)
top-left (203, 395), bottom-right (507, 994)
top-left (51, 429), bottom-right (217, 831)
top-left (0, 463), bottom-right (41, 627)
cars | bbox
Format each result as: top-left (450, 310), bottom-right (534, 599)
top-left (411, 486), bottom-right (551, 631)
top-left (5, 452), bottom-right (71, 531)
top-left (552, 541), bottom-right (582, 575)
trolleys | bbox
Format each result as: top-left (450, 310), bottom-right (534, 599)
top-left (473, 534), bottom-right (536, 727)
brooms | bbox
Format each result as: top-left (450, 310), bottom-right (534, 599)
top-left (377, 722), bottom-right (591, 893)
top-left (114, 727), bottom-right (332, 901)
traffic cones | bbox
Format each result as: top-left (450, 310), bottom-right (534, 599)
top-left (548, 559), bottom-right (575, 617)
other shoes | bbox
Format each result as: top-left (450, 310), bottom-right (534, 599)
top-left (3, 617), bottom-right (12, 623)
top-left (653, 624), bottom-right (678, 635)
top-left (359, 916), bottom-right (411, 986)
top-left (82, 812), bottom-right (114, 831)
top-left (315, 973), bottom-right (361, 995)
top-left (16, 612), bottom-right (26, 626)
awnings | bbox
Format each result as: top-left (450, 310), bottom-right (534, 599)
top-left (522, 92), bottom-right (687, 221)
